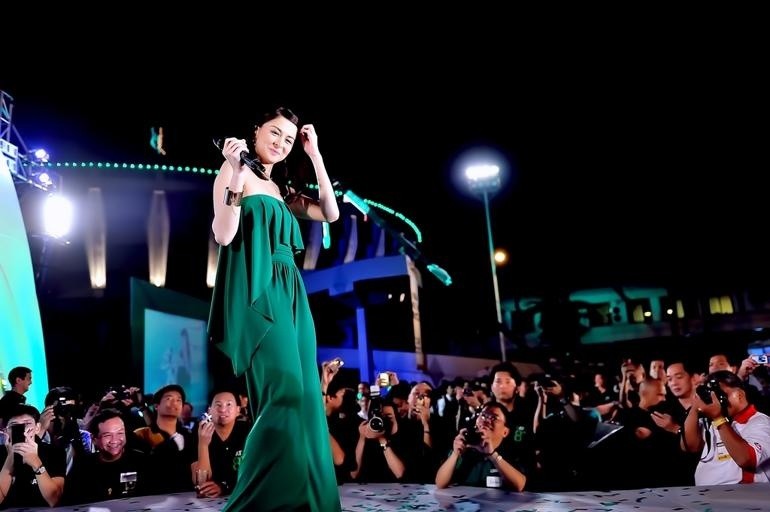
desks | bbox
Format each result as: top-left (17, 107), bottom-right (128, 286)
top-left (466, 161), bottom-right (510, 366)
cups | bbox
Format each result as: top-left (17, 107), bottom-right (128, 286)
top-left (476, 410), bottom-right (498, 422)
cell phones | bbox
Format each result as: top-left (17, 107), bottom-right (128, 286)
top-left (364, 401), bottom-right (384, 432)
top-left (113, 471), bottom-right (137, 498)
top-left (53, 387), bottom-right (75, 417)
top-left (696, 380), bottom-right (727, 406)
top-left (201, 410), bottom-right (212, 424)
top-left (538, 362), bottom-right (575, 395)
top-left (357, 383), bottom-right (366, 401)
top-left (463, 384), bottom-right (490, 396)
top-left (463, 416), bottom-right (484, 446)
top-left (752, 355), bottom-right (768, 364)
top-left (327, 357), bottom-right (345, 373)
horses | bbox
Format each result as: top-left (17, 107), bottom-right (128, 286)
top-left (370, 384), bottom-right (380, 397)
top-left (11, 423), bottom-right (25, 445)
top-left (380, 372), bottom-right (389, 387)
top-left (416, 395), bottom-right (424, 412)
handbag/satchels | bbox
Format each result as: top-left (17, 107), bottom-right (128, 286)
top-left (224, 184), bottom-right (247, 208)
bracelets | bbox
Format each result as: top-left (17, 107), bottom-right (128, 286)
top-left (675, 425), bottom-right (685, 437)
top-left (224, 184), bottom-right (247, 208)
top-left (494, 456), bottom-right (503, 464)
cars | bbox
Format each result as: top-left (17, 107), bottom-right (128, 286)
top-left (195, 469), bottom-right (209, 498)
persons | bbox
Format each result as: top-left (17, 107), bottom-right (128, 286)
top-left (206, 106), bottom-right (344, 512)
top-left (320, 351), bottom-right (769, 493)
top-left (0, 366), bottom-right (250, 510)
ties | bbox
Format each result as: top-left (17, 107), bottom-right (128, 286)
top-left (109, 383), bottom-right (130, 399)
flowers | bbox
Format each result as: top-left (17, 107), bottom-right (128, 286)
top-left (212, 135), bottom-right (265, 172)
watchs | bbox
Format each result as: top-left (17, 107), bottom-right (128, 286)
top-left (35, 465), bottom-right (46, 476)
top-left (711, 417), bottom-right (729, 430)
